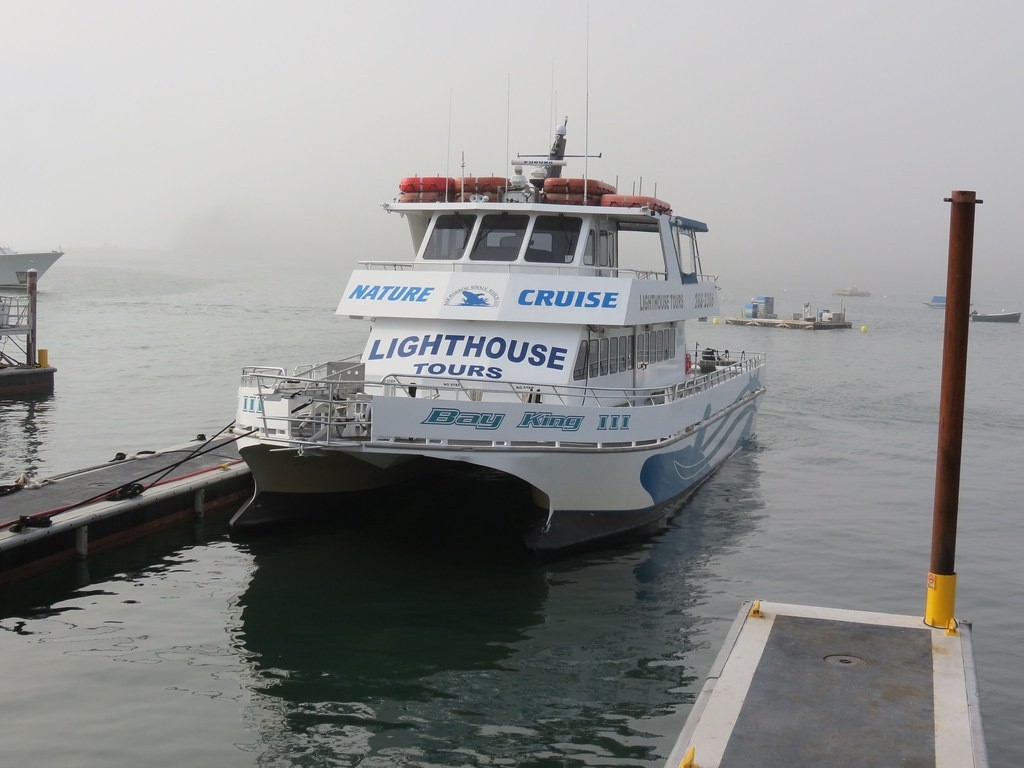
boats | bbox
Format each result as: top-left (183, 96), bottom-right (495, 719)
top-left (834, 286), bottom-right (870, 297)
top-left (226, 0), bottom-right (768, 569)
top-left (921, 294), bottom-right (975, 310)
top-left (971, 308), bottom-right (1022, 324)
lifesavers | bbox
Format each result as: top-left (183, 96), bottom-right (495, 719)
top-left (686, 354), bottom-right (691, 372)
top-left (398, 176), bottom-right (455, 203)
top-left (456, 177), bottom-right (511, 202)
top-left (542, 177), bottom-right (616, 206)
top-left (601, 192), bottom-right (672, 214)
